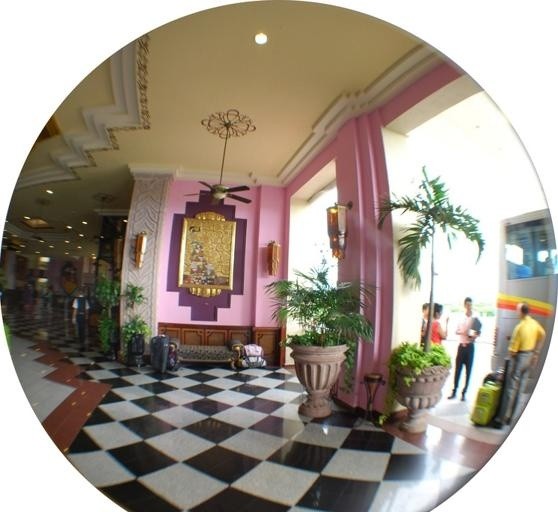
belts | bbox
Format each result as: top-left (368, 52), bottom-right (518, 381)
top-left (519, 349), bottom-right (536, 354)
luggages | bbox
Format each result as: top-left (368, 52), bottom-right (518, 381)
top-left (471, 358), bottom-right (510, 426)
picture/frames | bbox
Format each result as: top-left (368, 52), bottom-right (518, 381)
top-left (177, 217), bottom-right (237, 292)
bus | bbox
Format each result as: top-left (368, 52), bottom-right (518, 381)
top-left (491, 207), bottom-right (558, 394)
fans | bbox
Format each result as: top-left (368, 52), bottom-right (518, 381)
top-left (184, 121), bottom-right (255, 203)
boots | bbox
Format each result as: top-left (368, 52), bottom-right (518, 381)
top-left (448, 390), bottom-right (465, 401)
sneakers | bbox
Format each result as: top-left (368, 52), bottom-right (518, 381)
top-left (490, 421), bottom-right (503, 429)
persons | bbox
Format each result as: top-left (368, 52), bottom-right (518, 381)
top-left (447, 297), bottom-right (480, 402)
top-left (73, 290), bottom-right (90, 350)
top-left (492, 303), bottom-right (547, 428)
top-left (431, 304), bottom-right (450, 345)
top-left (420, 303), bottom-right (429, 344)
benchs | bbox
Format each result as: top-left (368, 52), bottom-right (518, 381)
top-left (169, 337), bottom-right (243, 372)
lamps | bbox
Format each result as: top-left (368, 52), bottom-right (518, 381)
top-left (134, 233), bottom-right (148, 268)
top-left (326, 203), bottom-right (349, 261)
top-left (267, 240), bottom-right (281, 277)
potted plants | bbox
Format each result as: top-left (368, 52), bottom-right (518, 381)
top-left (375, 163), bottom-right (490, 436)
top-left (120, 316), bottom-right (151, 369)
top-left (268, 266), bottom-right (376, 419)
